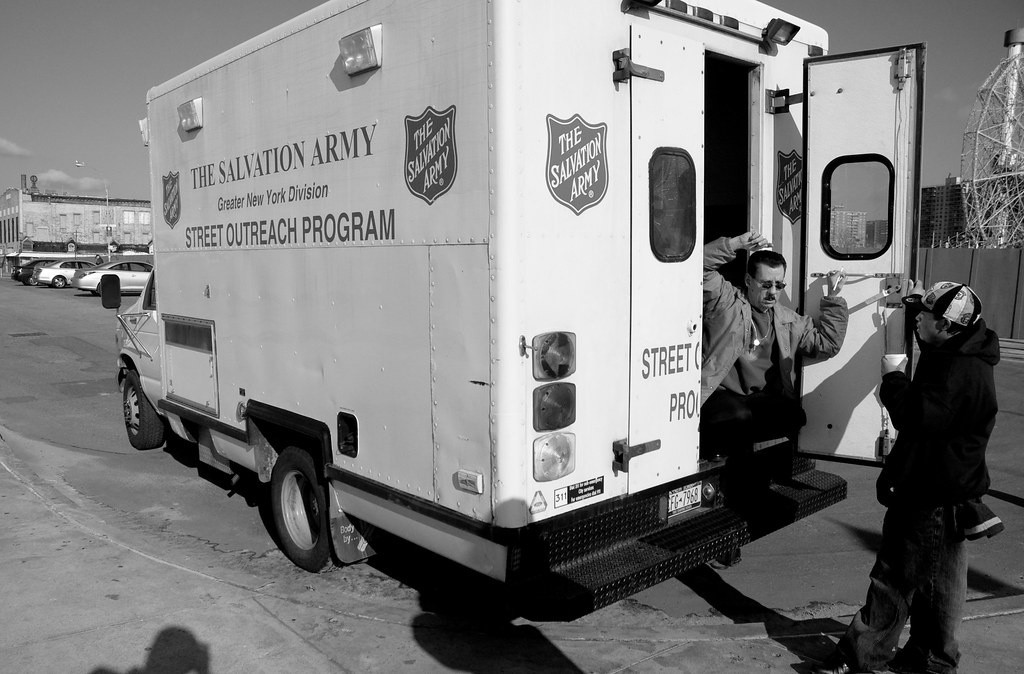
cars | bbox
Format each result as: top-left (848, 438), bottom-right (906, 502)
top-left (32, 260), bottom-right (97, 289)
top-left (71, 259), bottom-right (155, 295)
top-left (10, 258), bottom-right (59, 286)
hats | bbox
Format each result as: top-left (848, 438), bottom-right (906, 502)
top-left (901, 281), bottom-right (983, 327)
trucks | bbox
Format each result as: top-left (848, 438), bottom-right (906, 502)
top-left (97, 2), bottom-right (929, 623)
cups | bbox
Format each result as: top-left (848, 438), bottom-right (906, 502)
top-left (885, 354), bottom-right (906, 366)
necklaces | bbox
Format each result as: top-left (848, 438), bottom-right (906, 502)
top-left (751, 310), bottom-right (771, 352)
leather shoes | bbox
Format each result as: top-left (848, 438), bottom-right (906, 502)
top-left (808, 651), bottom-right (856, 674)
top-left (872, 657), bottom-right (910, 674)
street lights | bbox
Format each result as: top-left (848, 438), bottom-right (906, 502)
top-left (74, 163), bottom-right (112, 262)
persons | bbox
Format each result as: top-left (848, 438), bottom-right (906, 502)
top-left (95, 254), bottom-right (103, 265)
top-left (703, 231), bottom-right (848, 516)
top-left (814, 281), bottom-right (1004, 674)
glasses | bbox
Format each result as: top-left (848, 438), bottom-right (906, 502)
top-left (752, 276), bottom-right (786, 289)
